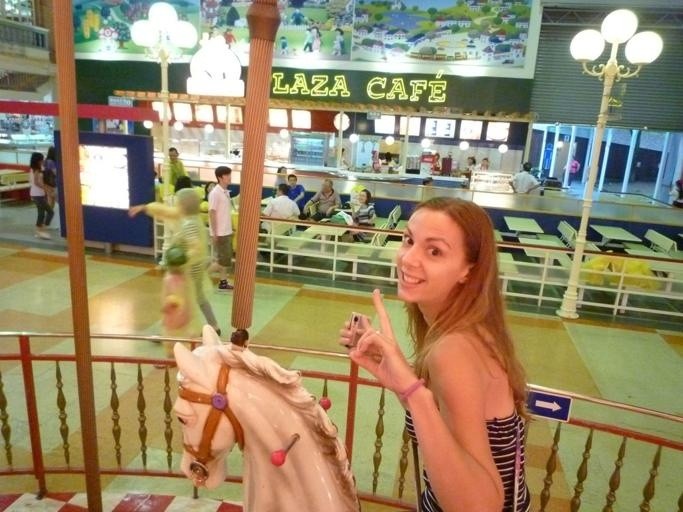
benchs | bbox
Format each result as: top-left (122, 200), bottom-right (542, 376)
top-left (260, 190), bottom-right (683, 316)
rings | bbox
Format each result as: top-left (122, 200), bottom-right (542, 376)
top-left (375, 330), bottom-right (381, 335)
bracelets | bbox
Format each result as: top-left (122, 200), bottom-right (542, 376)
top-left (398, 377), bottom-right (425, 401)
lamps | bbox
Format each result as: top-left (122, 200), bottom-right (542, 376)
top-left (385, 135), bottom-right (396, 146)
top-left (173, 121), bottom-right (184, 131)
top-left (498, 144), bottom-right (508, 154)
top-left (349, 134), bottom-right (359, 145)
top-left (421, 138), bottom-right (432, 148)
top-left (203, 124), bottom-right (214, 134)
top-left (143, 120), bottom-right (153, 130)
top-left (280, 129), bottom-right (288, 138)
top-left (459, 141), bottom-right (469, 150)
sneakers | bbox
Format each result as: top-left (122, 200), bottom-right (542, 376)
top-left (218, 281), bottom-right (233, 289)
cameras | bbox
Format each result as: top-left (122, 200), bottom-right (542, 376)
top-left (346, 312), bottom-right (372, 348)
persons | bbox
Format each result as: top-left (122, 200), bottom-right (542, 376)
top-left (341, 148), bottom-right (351, 168)
top-left (563, 156), bottom-right (581, 186)
top-left (421, 153), bottom-right (490, 186)
top-left (509, 162), bottom-right (541, 194)
top-left (340, 196), bottom-right (532, 512)
top-left (381, 152), bottom-right (396, 167)
top-left (30, 144), bottom-right (57, 240)
top-left (127, 146), bottom-right (237, 368)
top-left (369, 150), bottom-right (381, 170)
top-left (259, 166), bottom-right (341, 256)
top-left (349, 189), bottom-right (376, 241)
top-left (669, 179), bottom-right (683, 207)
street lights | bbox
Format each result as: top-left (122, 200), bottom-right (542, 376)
top-left (130, 3), bottom-right (197, 264)
top-left (553, 8), bottom-right (663, 320)
